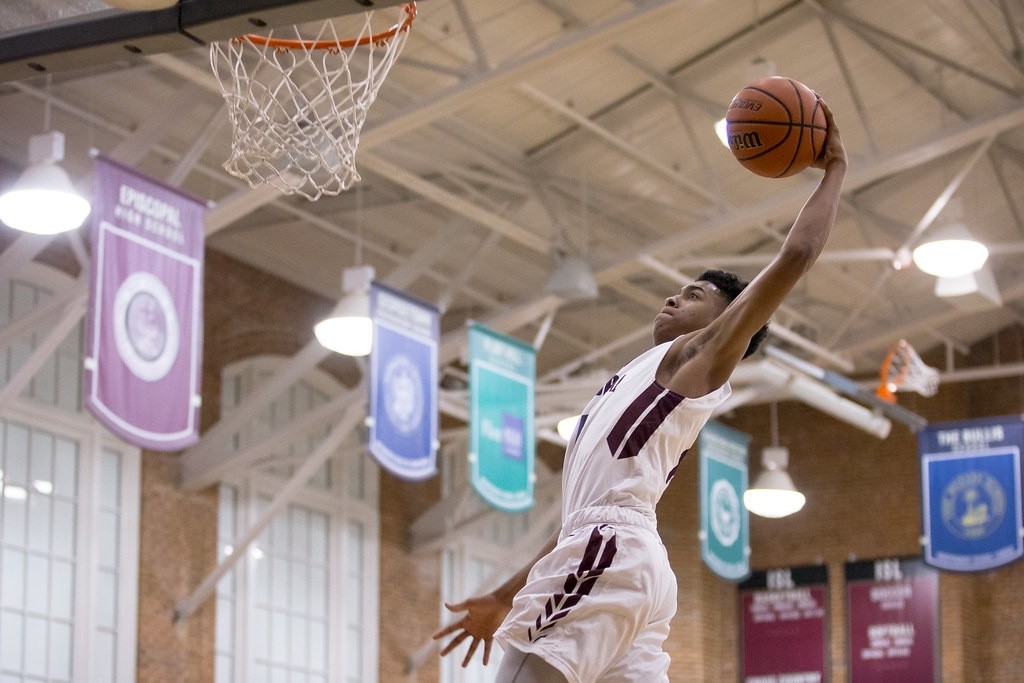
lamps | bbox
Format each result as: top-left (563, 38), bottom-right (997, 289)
top-left (313, 265), bottom-right (376, 357)
top-left (743, 443), bottom-right (806, 520)
top-left (914, 219), bottom-right (990, 277)
top-left (0, 131), bottom-right (91, 235)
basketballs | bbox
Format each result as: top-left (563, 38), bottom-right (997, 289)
top-left (729, 72), bottom-right (827, 180)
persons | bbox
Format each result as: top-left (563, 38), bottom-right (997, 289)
top-left (433, 89), bottom-right (847, 683)
